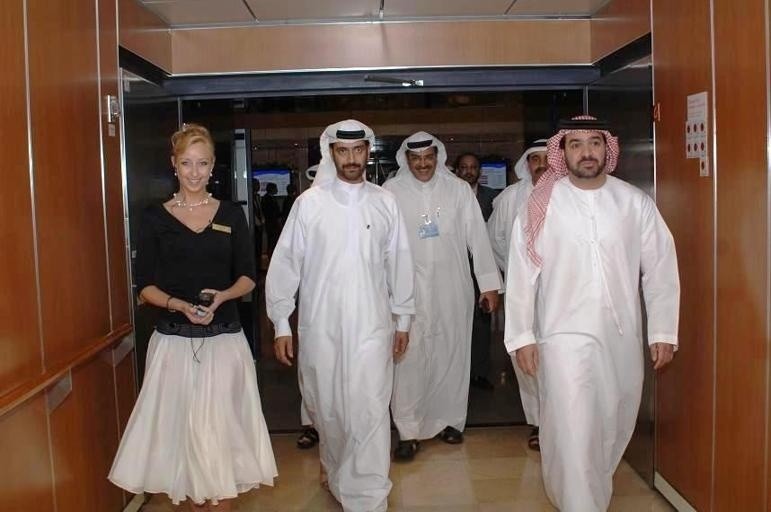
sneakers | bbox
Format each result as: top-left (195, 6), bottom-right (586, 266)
top-left (297, 428), bottom-right (316, 447)
top-left (529, 428), bottom-right (541, 450)
top-left (393, 439), bottom-right (418, 461)
top-left (444, 426), bottom-right (463, 443)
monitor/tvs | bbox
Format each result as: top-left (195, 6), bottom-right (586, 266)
top-left (250, 169), bottom-right (292, 198)
top-left (477, 161), bottom-right (509, 191)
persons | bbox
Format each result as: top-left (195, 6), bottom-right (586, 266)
top-left (251, 176), bottom-right (265, 245)
top-left (108, 121), bottom-right (284, 495)
top-left (379, 132), bottom-right (504, 462)
top-left (294, 390), bottom-right (320, 450)
top-left (263, 118), bottom-right (416, 509)
top-left (283, 184), bottom-right (296, 223)
top-left (260, 182), bottom-right (283, 257)
top-left (504, 115), bottom-right (680, 512)
top-left (487, 138), bottom-right (552, 452)
top-left (451, 149), bottom-right (500, 224)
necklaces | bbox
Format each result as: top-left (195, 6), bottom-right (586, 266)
top-left (172, 190), bottom-right (212, 208)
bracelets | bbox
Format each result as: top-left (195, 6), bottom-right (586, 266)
top-left (164, 296), bottom-right (176, 315)
